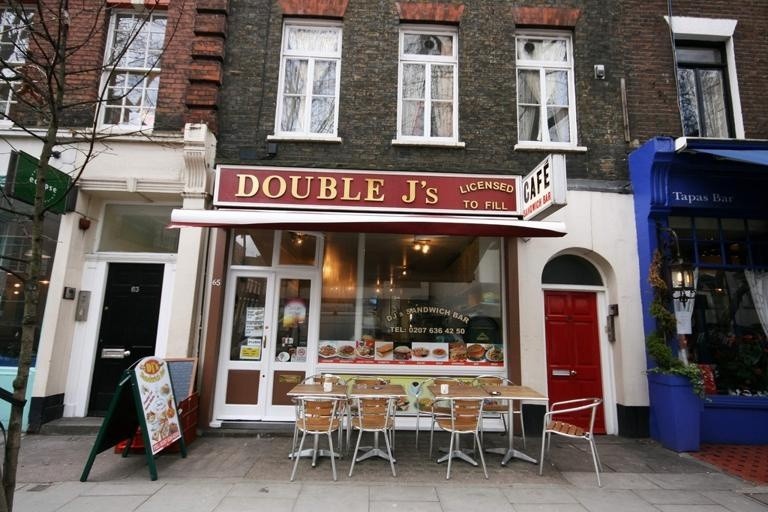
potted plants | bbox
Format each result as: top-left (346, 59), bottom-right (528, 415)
top-left (643, 246), bottom-right (707, 455)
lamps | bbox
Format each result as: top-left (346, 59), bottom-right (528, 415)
top-left (655, 218), bottom-right (698, 310)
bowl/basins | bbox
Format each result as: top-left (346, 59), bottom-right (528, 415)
top-left (141, 360), bottom-right (163, 376)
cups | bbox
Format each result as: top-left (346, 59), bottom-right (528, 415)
top-left (441, 383), bottom-right (449, 395)
top-left (356, 377), bottom-right (389, 391)
top-left (323, 372), bottom-right (333, 393)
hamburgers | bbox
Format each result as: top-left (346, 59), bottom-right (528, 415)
top-left (393, 346), bottom-right (411, 359)
top-left (467, 345), bottom-right (486, 360)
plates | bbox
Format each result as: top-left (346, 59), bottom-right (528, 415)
top-left (489, 392), bottom-right (501, 396)
top-left (319, 343), bottom-right (354, 359)
top-left (412, 347), bottom-right (447, 358)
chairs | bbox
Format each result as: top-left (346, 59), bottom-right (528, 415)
top-left (290, 374), bottom-right (409, 481)
top-left (472, 373), bottom-right (527, 451)
top-left (416, 378), bottom-right (467, 453)
top-left (430, 397), bottom-right (489, 480)
top-left (539, 397), bottom-right (603, 487)
